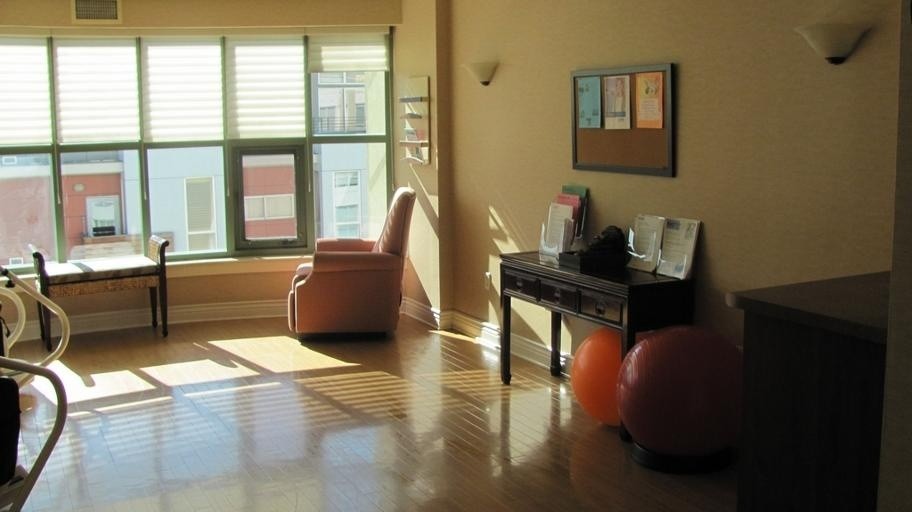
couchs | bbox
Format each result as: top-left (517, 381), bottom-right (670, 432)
top-left (285, 185), bottom-right (417, 345)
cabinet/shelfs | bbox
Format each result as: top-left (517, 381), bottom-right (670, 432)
top-left (719, 270), bottom-right (893, 512)
top-left (394, 74), bottom-right (431, 167)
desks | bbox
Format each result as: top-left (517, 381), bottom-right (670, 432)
top-left (492, 247), bottom-right (697, 443)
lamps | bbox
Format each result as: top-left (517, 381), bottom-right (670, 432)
top-left (791, 19), bottom-right (874, 68)
top-left (466, 61), bottom-right (501, 86)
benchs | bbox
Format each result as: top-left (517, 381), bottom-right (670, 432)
top-left (28, 233), bottom-right (171, 352)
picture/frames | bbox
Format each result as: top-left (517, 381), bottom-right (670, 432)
top-left (566, 61), bottom-right (679, 180)
